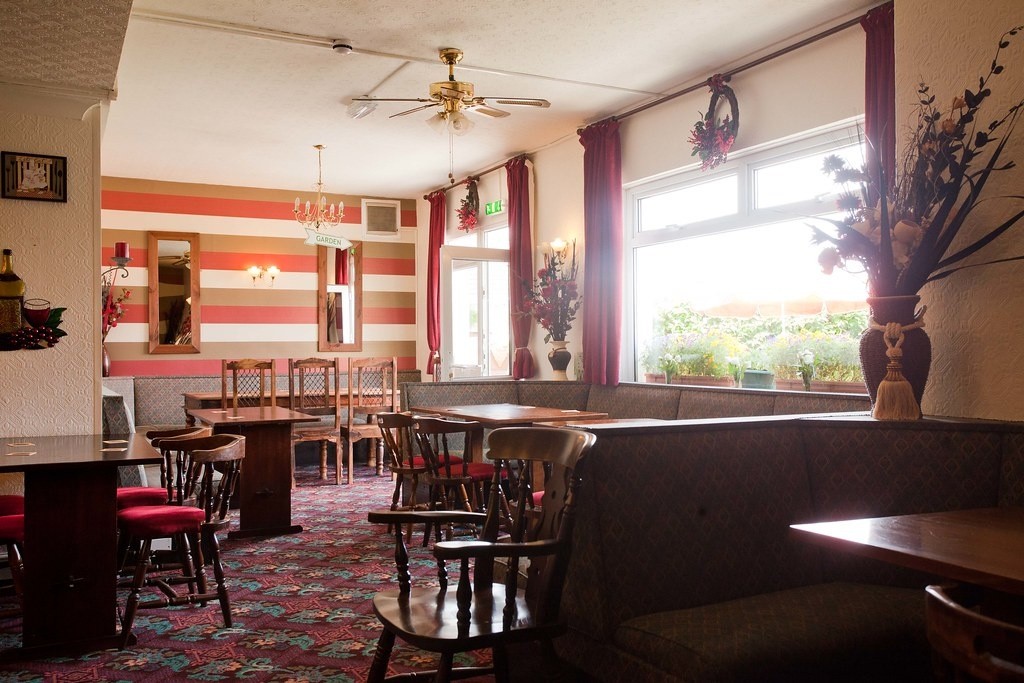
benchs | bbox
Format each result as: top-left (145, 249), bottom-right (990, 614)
top-left (399, 380), bottom-right (876, 439)
top-left (553, 420), bottom-right (1024, 683)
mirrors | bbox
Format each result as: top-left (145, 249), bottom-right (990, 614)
top-left (147, 231), bottom-right (200, 353)
top-left (319, 240), bottom-right (362, 352)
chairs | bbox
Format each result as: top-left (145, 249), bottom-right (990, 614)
top-left (366, 403), bottom-right (656, 683)
top-left (0, 426), bottom-right (246, 650)
top-left (222, 358), bottom-right (398, 484)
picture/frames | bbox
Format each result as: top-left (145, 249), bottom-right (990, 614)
top-left (2, 151), bottom-right (67, 203)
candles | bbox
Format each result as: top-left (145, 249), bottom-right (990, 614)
top-left (114, 242), bottom-right (130, 258)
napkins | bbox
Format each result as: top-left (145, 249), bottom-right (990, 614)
top-left (102, 440), bottom-right (129, 444)
top-left (228, 416), bottom-right (243, 419)
top-left (5, 452), bottom-right (36, 456)
top-left (8, 442), bottom-right (35, 446)
top-left (100, 447), bottom-right (127, 451)
top-left (211, 411), bottom-right (228, 413)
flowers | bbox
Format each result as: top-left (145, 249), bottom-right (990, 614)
top-left (810, 104), bottom-right (984, 296)
top-left (688, 110), bottom-right (735, 168)
top-left (455, 201), bottom-right (477, 232)
top-left (103, 280), bottom-right (130, 332)
top-left (522, 269), bottom-right (580, 341)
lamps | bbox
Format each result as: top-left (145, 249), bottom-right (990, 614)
top-left (247, 265), bottom-right (281, 286)
top-left (427, 111), bottom-right (474, 137)
top-left (293, 146), bottom-right (345, 229)
top-left (549, 239), bottom-right (567, 263)
top-left (349, 95), bottom-right (378, 121)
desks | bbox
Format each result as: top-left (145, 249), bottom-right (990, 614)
top-left (181, 387), bottom-right (400, 479)
top-left (787, 505), bottom-right (1024, 683)
top-left (409, 402), bottom-right (610, 532)
top-left (533, 417), bottom-right (677, 429)
top-left (187, 406), bottom-right (321, 539)
top-left (0, 432), bottom-right (163, 650)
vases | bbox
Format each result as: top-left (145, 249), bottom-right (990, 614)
top-left (103, 346), bottom-right (111, 376)
top-left (859, 295), bottom-right (931, 421)
top-left (548, 341), bottom-right (571, 381)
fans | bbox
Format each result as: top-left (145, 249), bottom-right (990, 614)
top-left (353, 48), bottom-right (551, 120)
